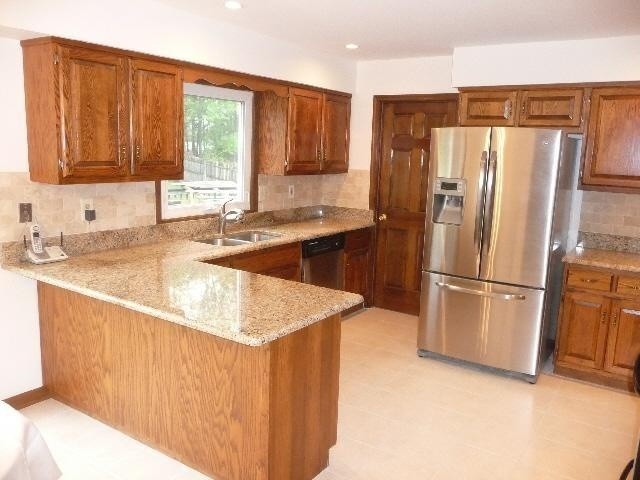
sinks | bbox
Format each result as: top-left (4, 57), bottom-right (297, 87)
top-left (228, 231), bottom-right (284, 243)
top-left (192, 238), bottom-right (248, 247)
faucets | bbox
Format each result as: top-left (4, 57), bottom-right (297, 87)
top-left (218, 198), bottom-right (245, 235)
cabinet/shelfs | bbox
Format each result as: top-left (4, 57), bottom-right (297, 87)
top-left (258, 86), bottom-right (350, 177)
top-left (208, 242), bottom-right (303, 285)
top-left (341, 226), bottom-right (378, 313)
top-left (369, 97), bottom-right (458, 219)
top-left (457, 85), bottom-right (588, 127)
top-left (377, 220), bottom-right (425, 313)
top-left (580, 81), bottom-right (640, 193)
top-left (552, 266), bottom-right (640, 397)
top-left (18, 36), bottom-right (183, 185)
top-left (301, 250), bottom-right (342, 291)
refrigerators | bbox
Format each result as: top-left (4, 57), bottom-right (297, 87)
top-left (416, 127), bottom-right (583, 385)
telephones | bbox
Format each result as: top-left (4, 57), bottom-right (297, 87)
top-left (24, 216), bottom-right (69, 264)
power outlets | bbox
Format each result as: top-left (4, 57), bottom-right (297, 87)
top-left (78, 197), bottom-right (98, 227)
